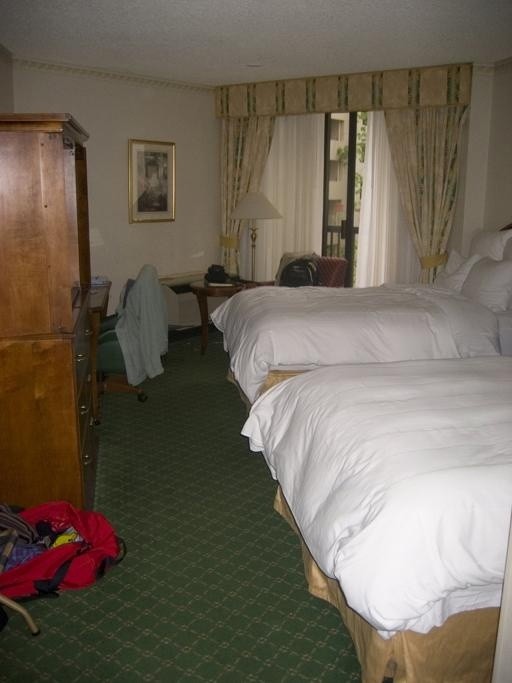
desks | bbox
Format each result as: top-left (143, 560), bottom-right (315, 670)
top-left (90, 281), bottom-right (112, 424)
top-left (190, 279), bottom-right (246, 354)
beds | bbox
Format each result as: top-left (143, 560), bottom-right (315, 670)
top-left (240, 353), bottom-right (512, 683)
top-left (209, 221), bottom-right (512, 413)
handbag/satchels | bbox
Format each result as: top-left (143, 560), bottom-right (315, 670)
top-left (0, 499), bottom-right (127, 601)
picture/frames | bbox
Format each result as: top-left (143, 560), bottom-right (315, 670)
top-left (127, 138), bottom-right (176, 224)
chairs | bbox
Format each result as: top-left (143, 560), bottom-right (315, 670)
top-left (275, 254), bottom-right (348, 288)
top-left (99, 263), bottom-right (177, 402)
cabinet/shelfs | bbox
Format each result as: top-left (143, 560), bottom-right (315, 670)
top-left (0, 111), bottom-right (99, 514)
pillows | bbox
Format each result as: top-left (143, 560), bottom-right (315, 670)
top-left (469, 225), bottom-right (512, 262)
top-left (502, 236), bottom-right (512, 264)
top-left (461, 256), bottom-right (512, 313)
top-left (433, 248), bottom-right (485, 292)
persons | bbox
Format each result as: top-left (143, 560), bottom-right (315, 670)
top-left (137, 162), bottom-right (167, 212)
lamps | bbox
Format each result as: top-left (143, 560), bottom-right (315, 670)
top-left (230, 191), bottom-right (284, 287)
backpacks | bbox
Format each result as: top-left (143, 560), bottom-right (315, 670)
top-left (280, 257), bottom-right (320, 287)
